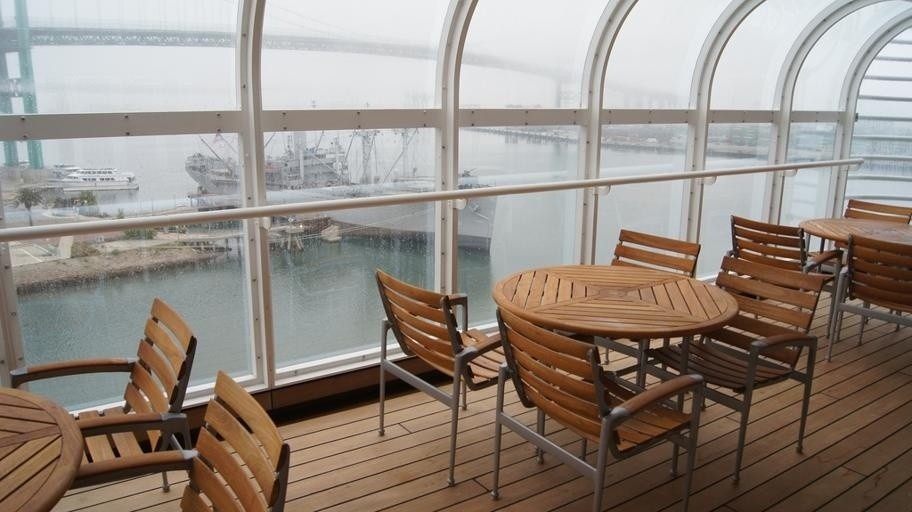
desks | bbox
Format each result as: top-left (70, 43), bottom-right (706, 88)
top-left (0, 384), bottom-right (84, 511)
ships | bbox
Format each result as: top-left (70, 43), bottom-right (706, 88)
top-left (183, 129), bottom-right (494, 252)
top-left (48, 165), bottom-right (138, 192)
